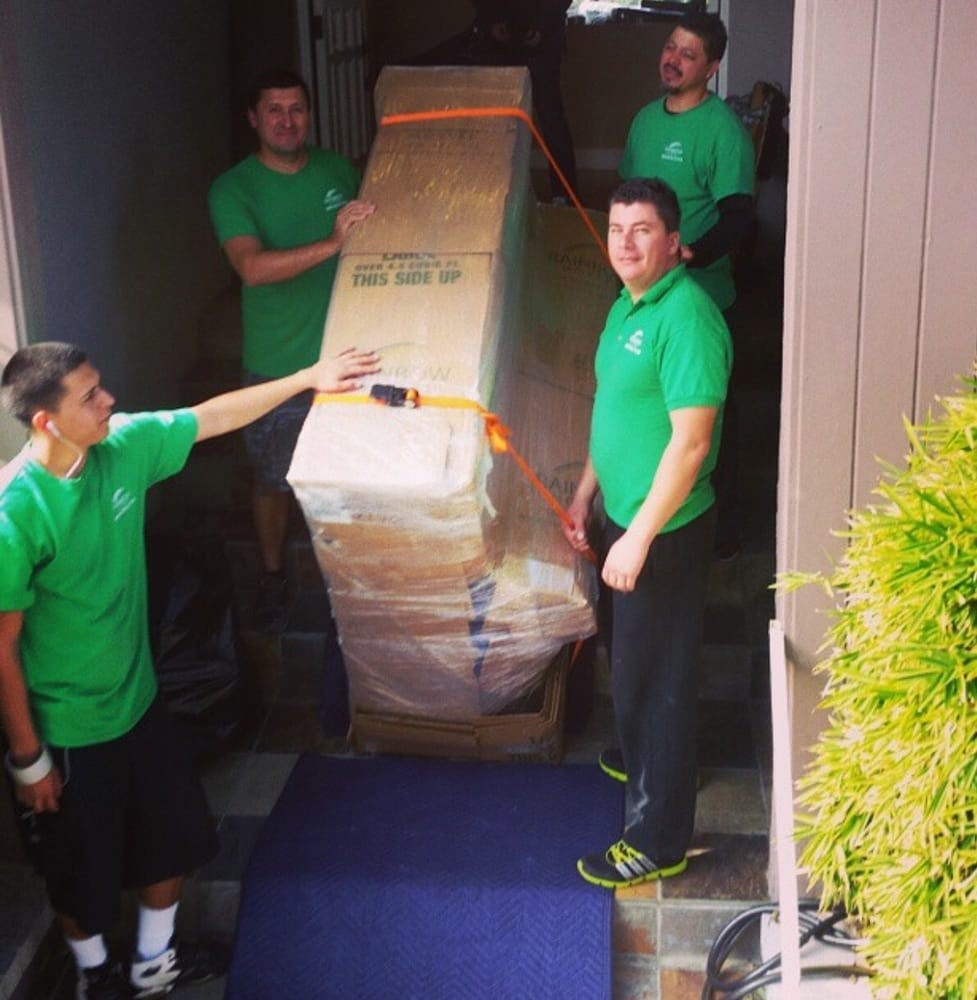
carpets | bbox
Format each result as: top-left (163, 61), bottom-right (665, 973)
top-left (223, 749), bottom-right (625, 1000)
top-left (319, 618), bottom-right (595, 736)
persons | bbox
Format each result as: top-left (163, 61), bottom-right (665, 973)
top-left (611, 13), bottom-right (757, 562)
top-left (404, 0), bottom-right (592, 210)
top-left (0, 342), bottom-right (375, 1000)
top-left (569, 177), bottom-right (732, 889)
top-left (208, 70), bottom-right (376, 633)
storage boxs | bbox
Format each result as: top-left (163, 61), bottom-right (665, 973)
top-left (286, 66), bottom-right (619, 723)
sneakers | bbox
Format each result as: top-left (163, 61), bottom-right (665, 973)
top-left (576, 839), bottom-right (688, 889)
top-left (256, 565), bottom-right (289, 633)
top-left (598, 749), bottom-right (701, 790)
top-left (74, 952), bottom-right (130, 1000)
top-left (127, 938), bottom-right (234, 1000)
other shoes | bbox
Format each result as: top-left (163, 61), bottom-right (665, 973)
top-left (716, 531), bottom-right (739, 562)
